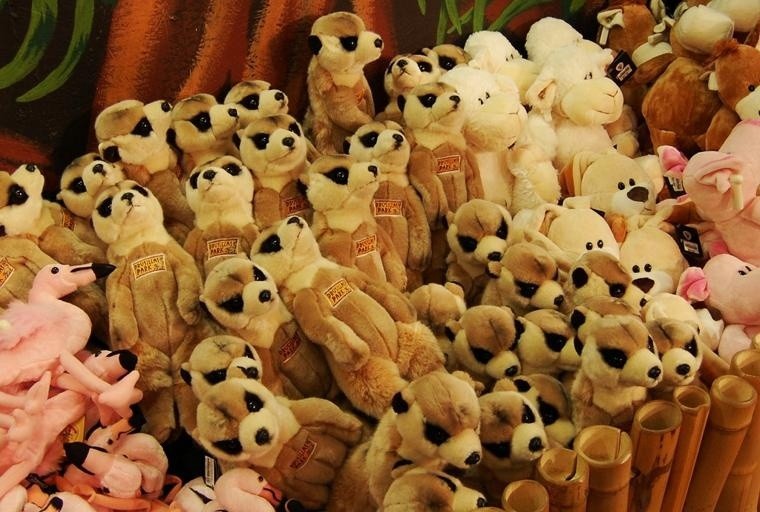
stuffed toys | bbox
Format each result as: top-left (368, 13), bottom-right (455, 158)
top-left (0, 0), bottom-right (760, 511)
top-left (1, 0), bottom-right (760, 510)
